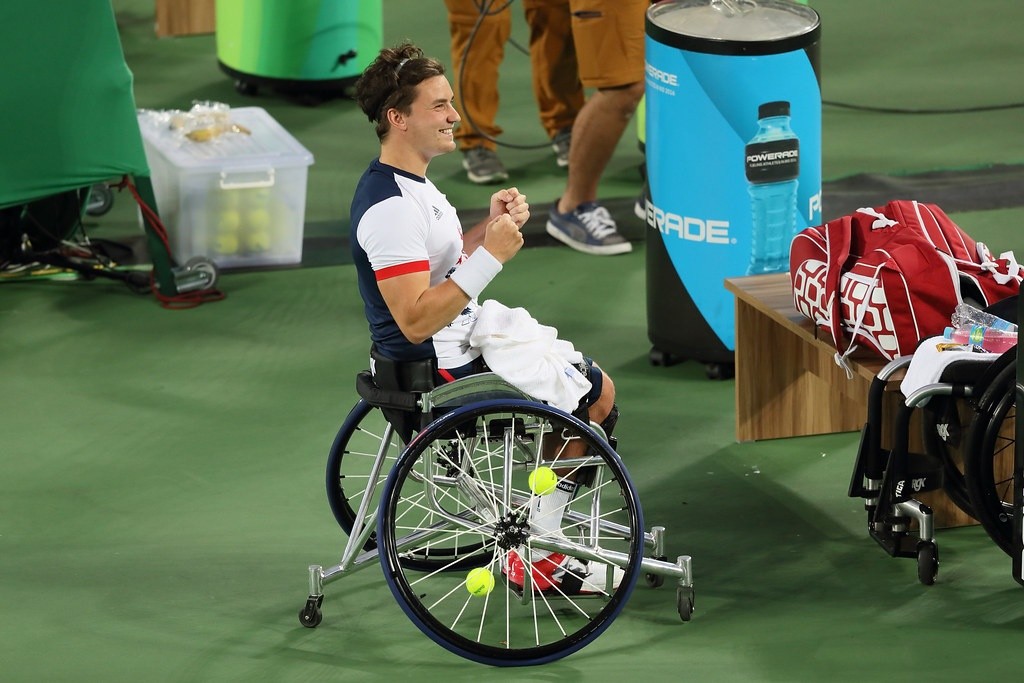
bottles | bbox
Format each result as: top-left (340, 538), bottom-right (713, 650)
top-left (744, 101), bottom-right (800, 279)
top-left (943, 322), bottom-right (1018, 353)
top-left (951, 305), bottom-right (1018, 335)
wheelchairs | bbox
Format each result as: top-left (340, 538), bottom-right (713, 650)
top-left (848, 280), bottom-right (1024, 592)
top-left (296, 342), bottom-right (699, 668)
top-left (1, 175), bottom-right (223, 300)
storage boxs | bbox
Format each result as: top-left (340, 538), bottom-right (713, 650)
top-left (137, 105), bottom-right (315, 272)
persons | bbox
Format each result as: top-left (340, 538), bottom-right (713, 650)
top-left (545, 0), bottom-right (659, 256)
top-left (442, 0), bottom-right (585, 185)
top-left (349, 40), bottom-right (629, 597)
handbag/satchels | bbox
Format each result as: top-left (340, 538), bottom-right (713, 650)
top-left (789, 199), bottom-right (1024, 374)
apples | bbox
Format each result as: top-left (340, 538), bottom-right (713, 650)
top-left (212, 184), bottom-right (270, 254)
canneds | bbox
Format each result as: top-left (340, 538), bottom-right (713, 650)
top-left (211, 0), bottom-right (384, 90)
top-left (642, 0), bottom-right (823, 367)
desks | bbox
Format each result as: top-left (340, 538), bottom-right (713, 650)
top-left (724, 272), bottom-right (1017, 531)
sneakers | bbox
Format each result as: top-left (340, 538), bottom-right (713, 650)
top-left (549, 127), bottom-right (573, 167)
top-left (500, 545), bottom-right (627, 595)
top-left (458, 146), bottom-right (508, 183)
top-left (545, 197), bottom-right (632, 255)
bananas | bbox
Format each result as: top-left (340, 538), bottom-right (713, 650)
top-left (168, 110), bottom-right (250, 141)
